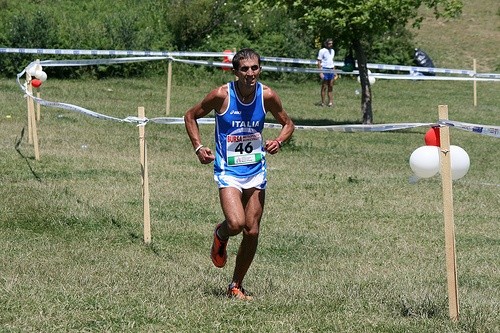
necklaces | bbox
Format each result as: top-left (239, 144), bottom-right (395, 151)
top-left (236, 81), bottom-right (256, 102)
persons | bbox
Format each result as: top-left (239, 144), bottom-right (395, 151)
top-left (184, 49), bottom-right (294, 300)
top-left (317, 38), bottom-right (337, 106)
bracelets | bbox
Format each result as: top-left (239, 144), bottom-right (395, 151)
top-left (276, 138), bottom-right (283, 147)
top-left (195, 144), bottom-right (203, 154)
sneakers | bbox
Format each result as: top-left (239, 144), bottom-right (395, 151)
top-left (227, 284), bottom-right (253, 299)
top-left (211, 224), bottom-right (227, 267)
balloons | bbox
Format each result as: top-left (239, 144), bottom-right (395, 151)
top-left (28, 63), bottom-right (47, 87)
top-left (222, 49), bottom-right (237, 71)
top-left (358, 69), bottom-right (375, 86)
top-left (409, 127), bottom-right (470, 180)
